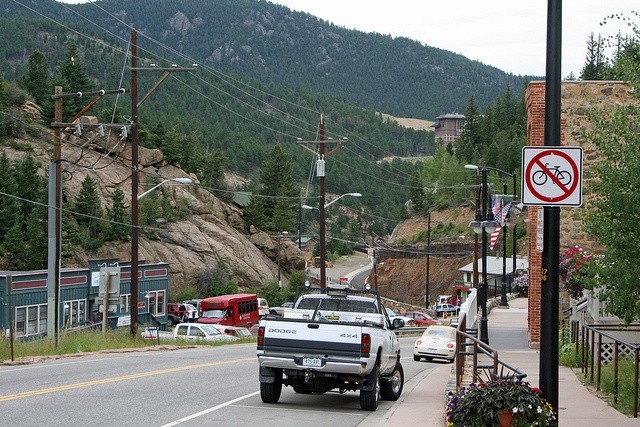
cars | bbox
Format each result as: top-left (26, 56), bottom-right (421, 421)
top-left (398, 311), bottom-right (442, 326)
top-left (432, 303), bottom-right (461, 317)
top-left (414, 325), bottom-right (456, 364)
top-left (270, 302), bottom-right (294, 316)
top-left (385, 308), bottom-right (414, 326)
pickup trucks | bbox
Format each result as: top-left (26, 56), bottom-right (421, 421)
top-left (141, 322), bottom-right (240, 343)
top-left (256, 288), bottom-right (404, 411)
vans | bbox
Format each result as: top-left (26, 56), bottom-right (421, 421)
top-left (257, 298), bottom-right (270, 318)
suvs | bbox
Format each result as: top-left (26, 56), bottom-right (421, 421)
top-left (183, 303), bottom-right (200, 321)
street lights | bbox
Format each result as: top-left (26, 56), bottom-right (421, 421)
top-left (490, 181), bottom-right (519, 310)
top-left (300, 192), bottom-right (363, 294)
top-left (130, 177), bottom-right (193, 336)
top-left (467, 165), bottom-right (501, 355)
top-left (465, 163), bottom-right (517, 275)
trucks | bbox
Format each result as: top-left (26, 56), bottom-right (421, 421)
top-left (184, 299), bottom-right (200, 310)
top-left (212, 325), bottom-right (252, 339)
top-left (168, 302), bottom-right (189, 327)
top-left (195, 293), bottom-right (260, 329)
